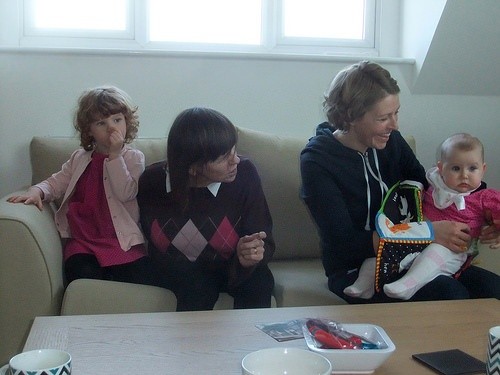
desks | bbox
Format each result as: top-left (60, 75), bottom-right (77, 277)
top-left (23, 298), bottom-right (500, 375)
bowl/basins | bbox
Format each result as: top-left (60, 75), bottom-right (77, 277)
top-left (301, 323), bottom-right (396, 374)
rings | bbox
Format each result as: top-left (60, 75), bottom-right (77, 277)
top-left (254, 248), bottom-right (257, 254)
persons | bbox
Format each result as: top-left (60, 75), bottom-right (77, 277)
top-left (343, 133), bottom-right (500, 298)
top-left (6, 84), bottom-right (160, 286)
top-left (136, 106), bottom-right (277, 311)
top-left (300, 59), bottom-right (500, 304)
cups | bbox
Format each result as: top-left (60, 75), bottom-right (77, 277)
top-left (9, 349), bottom-right (72, 375)
top-left (485, 326), bottom-right (500, 375)
top-left (241, 348), bottom-right (333, 375)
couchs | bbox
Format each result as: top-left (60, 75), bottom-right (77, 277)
top-left (0, 127), bottom-right (500, 367)
